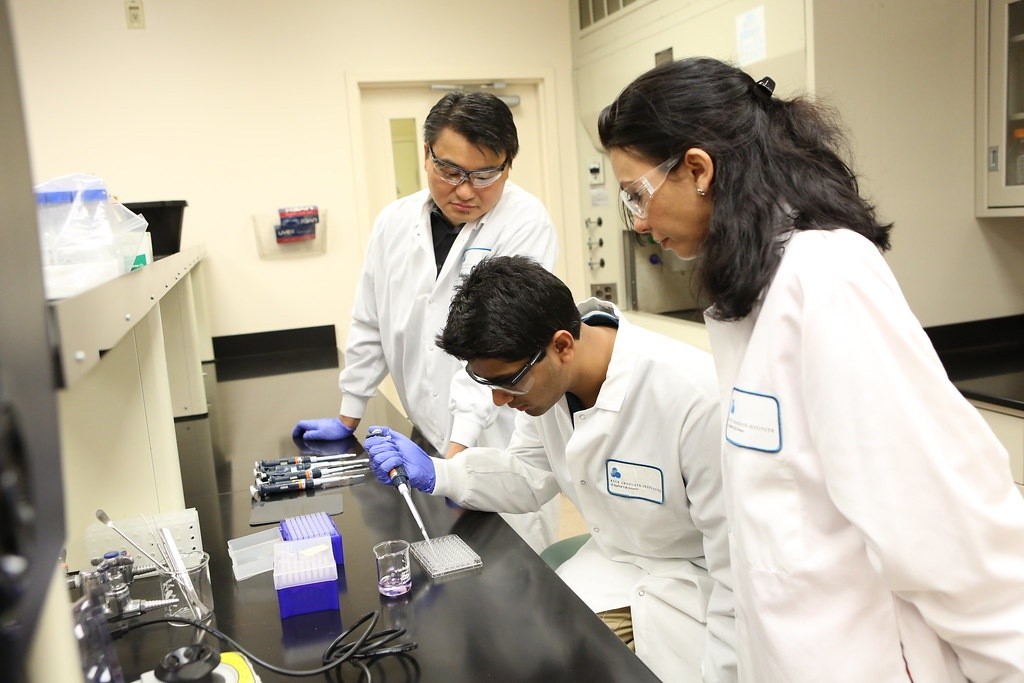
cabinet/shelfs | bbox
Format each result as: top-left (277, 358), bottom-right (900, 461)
top-left (974, 2), bottom-right (1024, 217)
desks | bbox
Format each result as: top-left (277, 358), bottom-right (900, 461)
top-left (70, 345), bottom-right (662, 683)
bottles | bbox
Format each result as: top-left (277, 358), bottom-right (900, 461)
top-left (1006, 128), bottom-right (1024, 185)
top-left (96, 552), bottom-right (136, 586)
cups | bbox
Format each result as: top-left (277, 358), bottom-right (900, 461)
top-left (372, 540), bottom-right (412, 597)
top-left (156, 550), bottom-right (217, 627)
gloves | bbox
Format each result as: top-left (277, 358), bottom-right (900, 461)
top-left (363, 426), bottom-right (435, 493)
top-left (293, 417), bottom-right (356, 441)
top-left (295, 439), bottom-right (352, 456)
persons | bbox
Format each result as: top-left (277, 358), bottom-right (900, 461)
top-left (293, 93), bottom-right (562, 555)
top-left (597, 57), bottom-right (1024, 683)
top-left (364, 254), bottom-right (737, 683)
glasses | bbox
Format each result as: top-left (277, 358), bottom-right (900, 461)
top-left (466, 349), bottom-right (546, 395)
top-left (621, 149), bottom-right (689, 219)
top-left (428, 141), bottom-right (509, 188)
